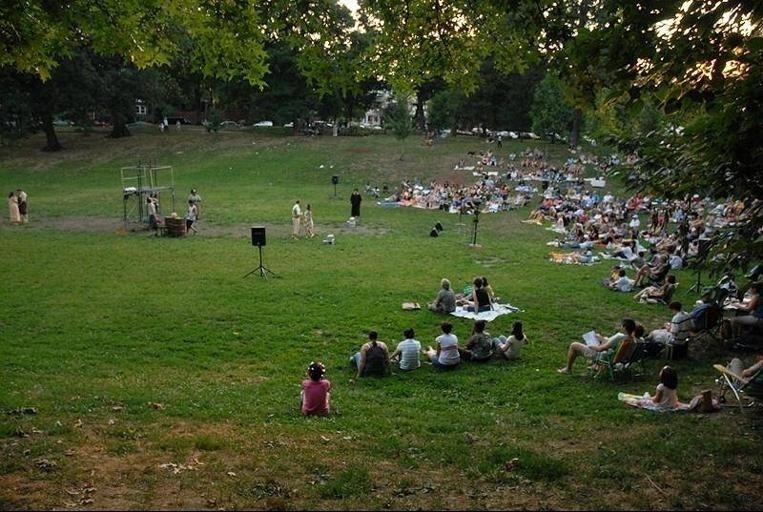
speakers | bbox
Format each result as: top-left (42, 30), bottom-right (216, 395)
top-left (252, 228), bottom-right (266, 246)
top-left (332, 176), bottom-right (338, 184)
top-left (431, 223), bottom-right (443, 237)
top-left (698, 237), bottom-right (712, 259)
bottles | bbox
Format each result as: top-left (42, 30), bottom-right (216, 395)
top-left (643, 392), bottom-right (650, 401)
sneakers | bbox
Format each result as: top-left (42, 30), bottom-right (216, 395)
top-left (557, 367), bottom-right (572, 375)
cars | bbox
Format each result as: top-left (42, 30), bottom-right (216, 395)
top-left (438, 126), bottom-right (599, 144)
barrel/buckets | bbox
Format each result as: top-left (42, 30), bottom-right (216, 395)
top-left (165, 216), bottom-right (186, 237)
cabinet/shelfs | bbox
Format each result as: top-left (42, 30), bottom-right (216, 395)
top-left (120, 164), bottom-right (175, 222)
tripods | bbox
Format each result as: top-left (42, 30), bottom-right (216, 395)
top-left (242, 246), bottom-right (280, 280)
top-left (686, 258), bottom-right (706, 295)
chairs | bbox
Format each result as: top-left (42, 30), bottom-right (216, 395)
top-left (592, 237), bottom-right (763, 415)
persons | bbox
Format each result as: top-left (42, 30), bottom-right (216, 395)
top-left (8, 192), bottom-right (20, 225)
top-left (300, 276), bottom-right (528, 415)
top-left (350, 188), bottom-right (363, 223)
top-left (561, 297), bottom-right (763, 411)
top-left (303, 204), bottom-right (315, 238)
top-left (292, 201), bottom-right (304, 241)
top-left (148, 185), bottom-right (202, 236)
top-left (16, 189), bottom-right (29, 224)
top-left (158, 116), bottom-right (182, 136)
top-left (367, 122), bottom-right (763, 306)
